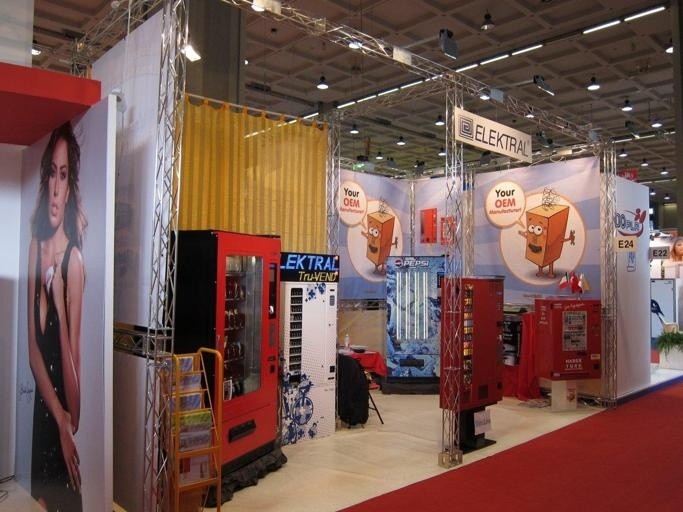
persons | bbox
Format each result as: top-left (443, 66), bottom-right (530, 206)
top-left (25, 121), bottom-right (89, 510)
top-left (669, 238), bottom-right (683, 261)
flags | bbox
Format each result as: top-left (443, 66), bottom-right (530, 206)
top-left (559, 270), bottom-right (591, 293)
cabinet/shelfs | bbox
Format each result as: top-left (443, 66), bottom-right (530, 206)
top-left (157, 347), bottom-right (224, 512)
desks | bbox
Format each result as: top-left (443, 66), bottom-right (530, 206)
top-left (338, 349), bottom-right (380, 390)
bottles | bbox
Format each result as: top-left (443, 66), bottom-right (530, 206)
top-left (344, 333), bottom-right (351, 352)
top-left (224, 258), bottom-right (244, 399)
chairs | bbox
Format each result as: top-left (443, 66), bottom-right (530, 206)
top-left (337, 353), bottom-right (384, 429)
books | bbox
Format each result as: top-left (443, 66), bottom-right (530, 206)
top-left (166, 374), bottom-right (201, 391)
top-left (164, 357), bottom-right (193, 375)
top-left (172, 411), bottom-right (212, 431)
top-left (179, 422), bottom-right (209, 450)
top-left (168, 393), bottom-right (201, 411)
top-left (179, 454), bottom-right (208, 483)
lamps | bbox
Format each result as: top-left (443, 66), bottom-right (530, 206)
top-left (625, 120), bottom-right (640, 140)
top-left (533, 74), bottom-right (554, 97)
top-left (651, 116), bottom-right (662, 128)
top-left (664, 192), bottom-right (670, 200)
top-left (375, 152), bottom-right (384, 160)
top-left (396, 136), bottom-right (405, 146)
top-left (435, 115), bottom-right (445, 126)
top-left (622, 99), bottom-right (632, 112)
top-left (414, 160), bottom-right (425, 168)
top-left (666, 38), bottom-right (673, 53)
top-left (439, 29), bottom-right (458, 60)
top-left (618, 148), bottom-right (627, 158)
top-left (317, 75), bottom-right (329, 90)
top-left (437, 147), bottom-right (446, 156)
top-left (649, 189), bottom-right (656, 196)
top-left (640, 158), bottom-right (648, 167)
top-left (350, 125), bottom-right (359, 135)
top-left (661, 167), bottom-right (669, 175)
top-left (586, 77), bottom-right (600, 91)
top-left (480, 12), bottom-right (495, 31)
